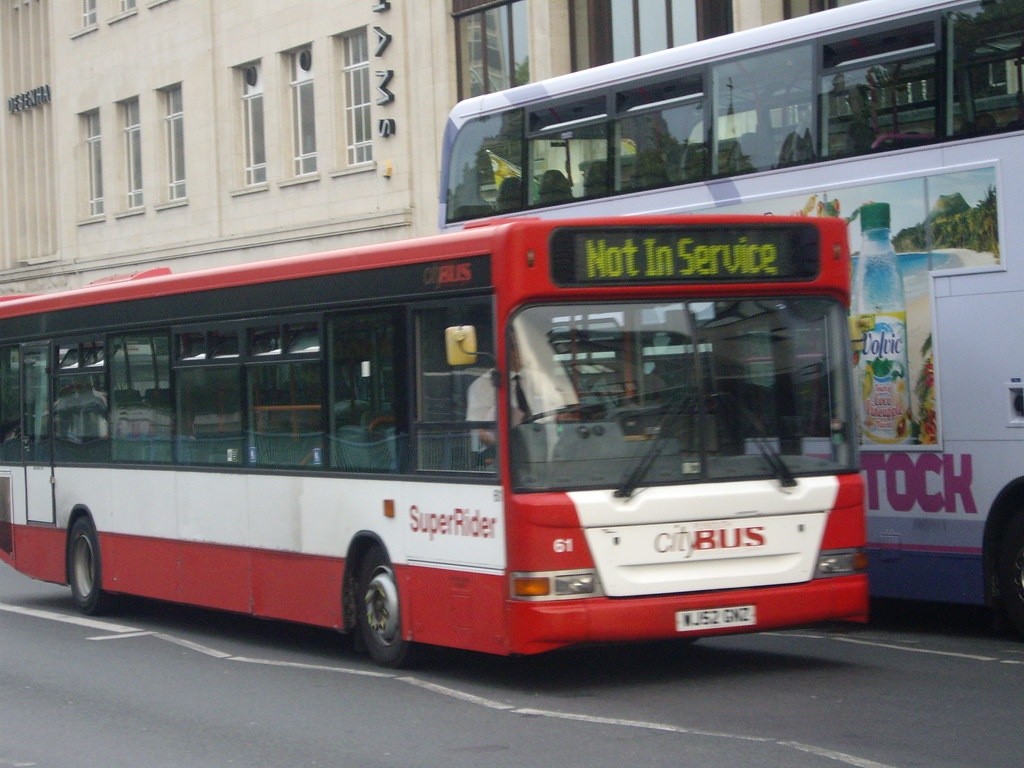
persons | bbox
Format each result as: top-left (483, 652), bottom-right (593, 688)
top-left (222, 364), bottom-right (284, 433)
top-left (465, 326), bottom-right (538, 471)
top-left (582, 161), bottom-right (609, 200)
top-left (683, 140), bottom-right (707, 184)
top-left (631, 148), bottom-right (672, 191)
top-left (718, 127), bottom-right (812, 176)
top-left (497, 176), bottom-right (525, 215)
top-left (537, 170), bottom-right (574, 205)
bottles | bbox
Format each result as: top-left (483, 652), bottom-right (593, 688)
top-left (849, 202), bottom-right (914, 446)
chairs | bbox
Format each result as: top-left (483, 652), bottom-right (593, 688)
top-left (452, 140), bottom-right (711, 222)
top-left (109, 375), bottom-right (361, 430)
top-left (713, 380), bottom-right (798, 458)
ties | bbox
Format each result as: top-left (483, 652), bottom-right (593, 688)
top-left (513, 375), bottom-right (533, 423)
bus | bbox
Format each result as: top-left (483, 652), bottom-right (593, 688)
top-left (0, 213), bottom-right (871, 671)
top-left (436, 1), bottom-right (1024, 647)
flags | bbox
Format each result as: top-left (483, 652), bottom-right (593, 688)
top-left (486, 149), bottom-right (552, 204)
